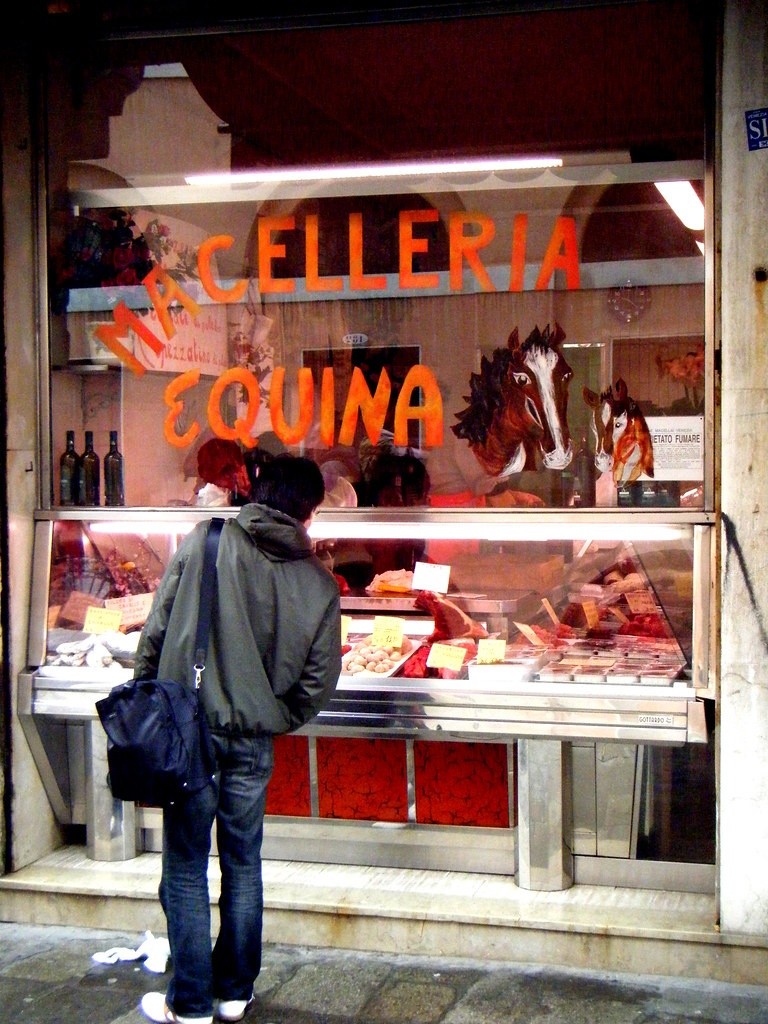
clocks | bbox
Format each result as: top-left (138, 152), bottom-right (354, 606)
top-left (607, 277), bottom-right (652, 324)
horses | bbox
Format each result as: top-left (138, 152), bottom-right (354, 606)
top-left (583, 378), bottom-right (654, 489)
top-left (449, 323), bottom-right (573, 478)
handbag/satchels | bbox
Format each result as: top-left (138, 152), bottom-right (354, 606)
top-left (95, 676), bottom-right (218, 818)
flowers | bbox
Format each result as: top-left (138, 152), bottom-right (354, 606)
top-left (50, 529), bottom-right (161, 605)
top-left (55, 210), bottom-right (201, 318)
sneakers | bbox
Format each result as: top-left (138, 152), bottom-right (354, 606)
top-left (218, 992), bottom-right (254, 1021)
top-left (141, 992), bottom-right (213, 1024)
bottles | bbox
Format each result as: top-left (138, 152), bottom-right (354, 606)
top-left (78, 431), bottom-right (100, 506)
top-left (104, 431), bottom-right (123, 507)
top-left (59, 430), bottom-right (79, 505)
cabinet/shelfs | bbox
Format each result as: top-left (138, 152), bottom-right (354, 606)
top-left (11, 150), bottom-right (718, 898)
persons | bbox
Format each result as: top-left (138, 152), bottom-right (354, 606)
top-left (304, 422), bottom-right (362, 510)
top-left (421, 427), bottom-right (544, 507)
top-left (136, 452), bottom-right (344, 1024)
